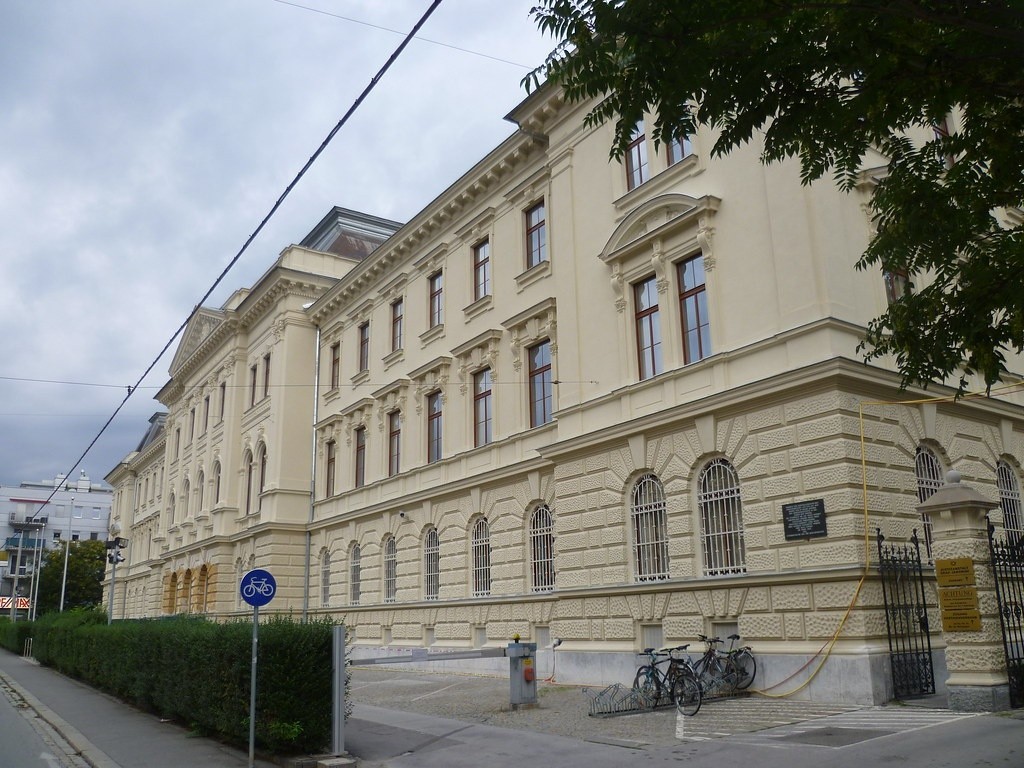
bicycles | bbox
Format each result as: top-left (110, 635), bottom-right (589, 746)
top-left (632, 633), bottom-right (757, 716)
